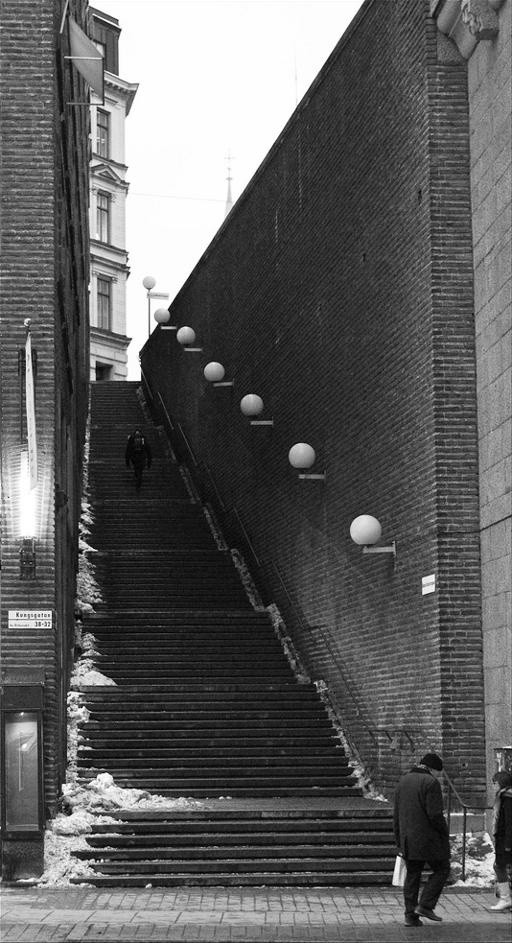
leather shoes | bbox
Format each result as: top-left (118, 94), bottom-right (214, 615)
top-left (404, 918), bottom-right (423, 926)
top-left (414, 905), bottom-right (442, 921)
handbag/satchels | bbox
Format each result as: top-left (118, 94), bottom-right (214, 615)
top-left (391, 852), bottom-right (407, 887)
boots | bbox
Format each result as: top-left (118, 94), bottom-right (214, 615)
top-left (490, 880), bottom-right (511, 911)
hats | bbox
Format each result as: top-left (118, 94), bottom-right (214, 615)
top-left (420, 753), bottom-right (443, 770)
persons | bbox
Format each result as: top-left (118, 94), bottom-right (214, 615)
top-left (489, 771), bottom-right (512, 911)
top-left (125, 429), bottom-right (153, 493)
top-left (393, 753), bottom-right (451, 927)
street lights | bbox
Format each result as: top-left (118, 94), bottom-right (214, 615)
top-left (142, 274), bottom-right (156, 336)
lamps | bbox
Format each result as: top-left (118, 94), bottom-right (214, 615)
top-left (204, 361), bottom-right (236, 387)
top-left (240, 393), bottom-right (274, 428)
top-left (287, 443), bottom-right (327, 481)
top-left (350, 514), bottom-right (397, 555)
top-left (154, 307), bottom-right (205, 352)
